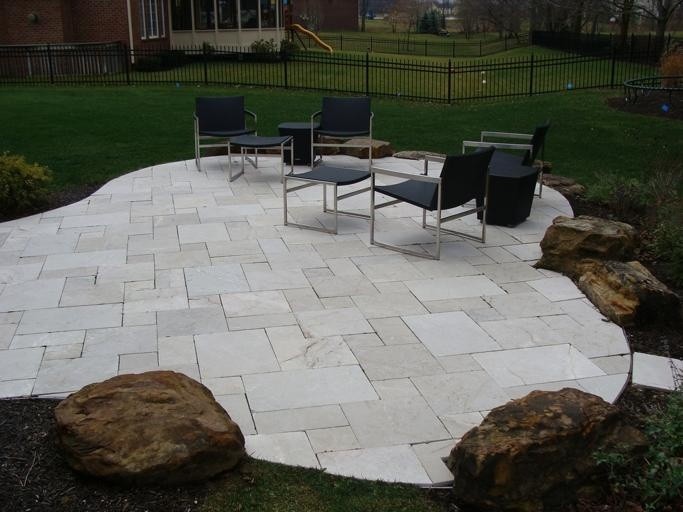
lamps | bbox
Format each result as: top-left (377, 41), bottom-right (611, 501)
top-left (27, 13), bottom-right (38, 23)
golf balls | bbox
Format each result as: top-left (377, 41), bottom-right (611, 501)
top-left (482, 80), bottom-right (486, 84)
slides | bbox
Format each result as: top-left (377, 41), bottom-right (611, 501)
top-left (290, 23), bottom-right (333, 53)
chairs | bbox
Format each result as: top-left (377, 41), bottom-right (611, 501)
top-left (462, 119), bottom-right (551, 207)
top-left (370, 145), bottom-right (496, 261)
top-left (193, 96), bottom-right (258, 171)
top-left (310, 97), bottom-right (374, 171)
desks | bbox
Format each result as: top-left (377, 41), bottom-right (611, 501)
top-left (476, 166), bottom-right (541, 228)
top-left (278, 122), bottom-right (320, 165)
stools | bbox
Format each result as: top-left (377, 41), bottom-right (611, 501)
top-left (228, 134), bottom-right (293, 183)
top-left (283, 165), bottom-right (372, 235)
